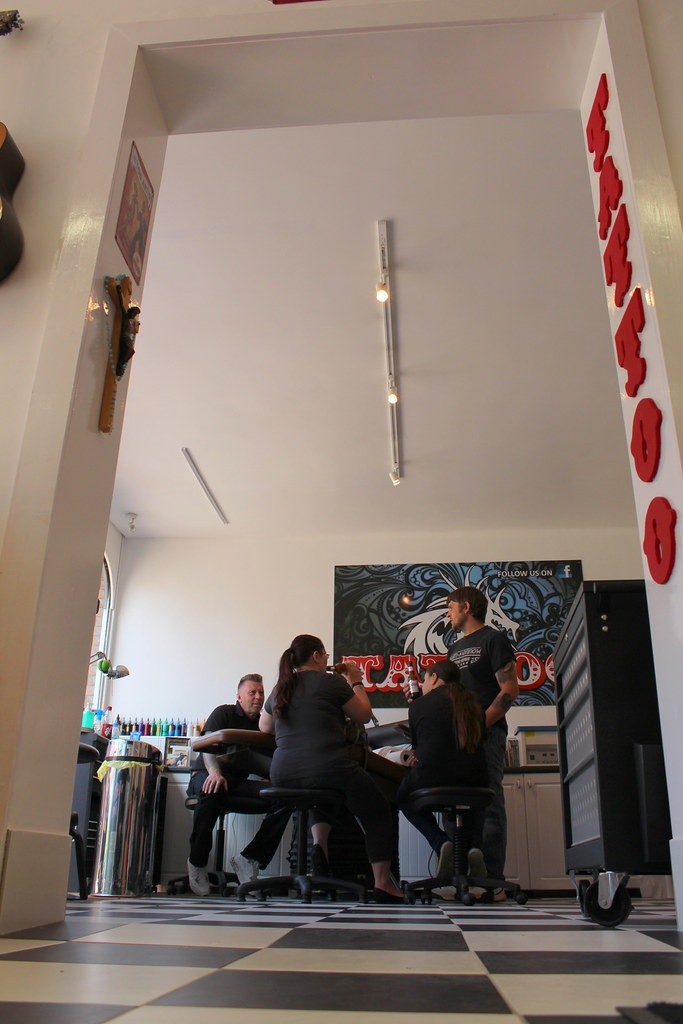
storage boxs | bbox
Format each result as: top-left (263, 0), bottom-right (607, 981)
top-left (513, 724), bottom-right (560, 766)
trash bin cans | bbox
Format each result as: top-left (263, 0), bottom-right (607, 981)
top-left (91, 738), bottom-right (163, 898)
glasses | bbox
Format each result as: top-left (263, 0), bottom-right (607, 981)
top-left (320, 653), bottom-right (329, 659)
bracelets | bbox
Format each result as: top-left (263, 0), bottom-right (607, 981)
top-left (352, 682), bottom-right (363, 689)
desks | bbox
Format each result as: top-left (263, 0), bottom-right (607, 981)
top-left (190, 728), bottom-right (273, 754)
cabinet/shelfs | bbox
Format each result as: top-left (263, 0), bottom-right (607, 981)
top-left (163, 773), bottom-right (280, 894)
top-left (67, 732), bottom-right (110, 901)
top-left (119, 734), bottom-right (187, 772)
top-left (524, 765), bottom-right (639, 890)
top-left (401, 772), bottom-right (525, 892)
top-left (281, 809), bottom-right (405, 881)
top-left (552, 579), bottom-right (672, 880)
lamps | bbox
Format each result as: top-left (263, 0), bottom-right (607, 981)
top-left (375, 269), bottom-right (389, 302)
top-left (387, 374), bottom-right (400, 403)
top-left (126, 512), bottom-right (137, 533)
top-left (389, 465), bottom-right (400, 486)
top-left (89, 651), bottom-right (130, 681)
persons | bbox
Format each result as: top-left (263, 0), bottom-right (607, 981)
top-left (396, 587), bottom-right (520, 901)
top-left (188, 635), bottom-right (409, 904)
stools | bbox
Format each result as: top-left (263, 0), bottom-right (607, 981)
top-left (68, 739), bottom-right (101, 901)
top-left (166, 793), bottom-right (240, 902)
top-left (233, 786), bottom-right (369, 905)
top-left (405, 782), bottom-right (529, 907)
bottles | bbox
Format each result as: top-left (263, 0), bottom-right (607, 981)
top-left (407, 661), bottom-right (420, 698)
top-left (101, 705), bottom-right (114, 740)
top-left (326, 663), bottom-right (347, 674)
top-left (111, 714), bottom-right (206, 740)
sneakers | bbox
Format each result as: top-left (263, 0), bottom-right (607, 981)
top-left (229, 854), bottom-right (261, 897)
top-left (187, 857), bottom-right (210, 896)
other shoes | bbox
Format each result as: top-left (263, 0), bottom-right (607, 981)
top-left (466, 846), bottom-right (487, 878)
top-left (472, 886), bottom-right (507, 901)
top-left (311, 844), bottom-right (329, 877)
top-left (432, 887), bottom-right (472, 900)
top-left (373, 888), bottom-right (404, 904)
top-left (435, 839), bottom-right (454, 879)
